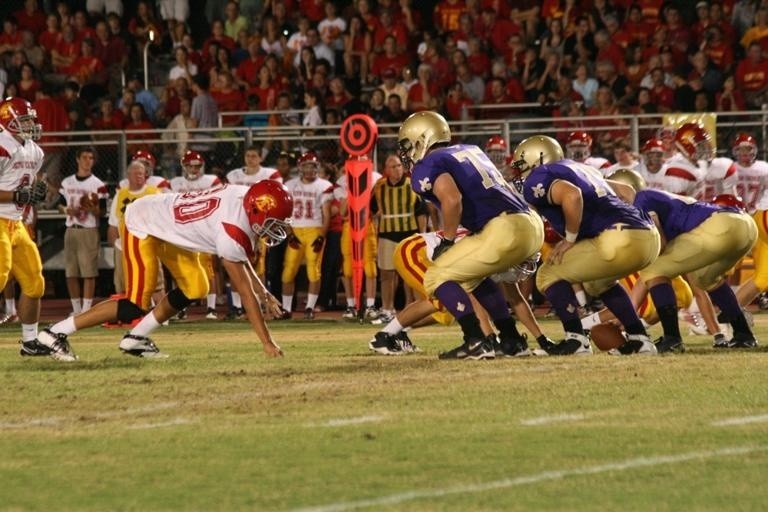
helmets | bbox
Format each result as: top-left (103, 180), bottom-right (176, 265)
top-left (566, 132), bottom-right (593, 148)
top-left (0, 96), bottom-right (42, 141)
top-left (675, 123), bottom-right (712, 161)
top-left (511, 135), bottom-right (563, 194)
top-left (399, 110), bottom-right (451, 168)
top-left (733, 135), bottom-right (758, 160)
top-left (297, 152), bottom-right (320, 179)
top-left (640, 139), bottom-right (669, 162)
top-left (181, 151), bottom-right (204, 179)
top-left (608, 167), bottom-right (648, 192)
top-left (133, 151), bottom-right (155, 175)
top-left (486, 137), bottom-right (507, 162)
top-left (244, 179), bottom-right (292, 247)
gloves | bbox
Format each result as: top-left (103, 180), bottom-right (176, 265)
top-left (287, 232), bottom-right (301, 250)
top-left (432, 238), bottom-right (454, 261)
top-left (311, 236), bottom-right (324, 252)
top-left (14, 181), bottom-right (48, 205)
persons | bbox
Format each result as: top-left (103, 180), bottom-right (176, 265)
top-left (0, 0), bottom-right (768, 360)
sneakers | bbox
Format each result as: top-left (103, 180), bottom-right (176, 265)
top-left (369, 333), bottom-right (423, 357)
top-left (342, 305), bottom-right (398, 324)
top-left (0, 314), bottom-right (19, 326)
top-left (304, 308), bottom-right (315, 320)
top-left (487, 332), bottom-right (504, 356)
top-left (532, 329), bottom-right (593, 357)
top-left (206, 306), bottom-right (293, 320)
top-left (118, 333), bottom-right (169, 357)
top-left (439, 336), bottom-right (495, 359)
top-left (713, 338), bottom-right (759, 349)
top-left (541, 339), bottom-right (560, 356)
top-left (608, 332), bottom-right (657, 356)
top-left (677, 308), bottom-right (709, 335)
top-left (653, 336), bottom-right (685, 354)
top-left (19, 329), bottom-right (79, 362)
top-left (500, 333), bottom-right (532, 357)
top-left (712, 333), bottom-right (726, 345)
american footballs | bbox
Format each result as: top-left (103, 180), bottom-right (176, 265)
top-left (591, 325), bottom-right (625, 351)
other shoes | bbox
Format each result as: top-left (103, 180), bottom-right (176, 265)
top-left (583, 329), bottom-right (592, 341)
